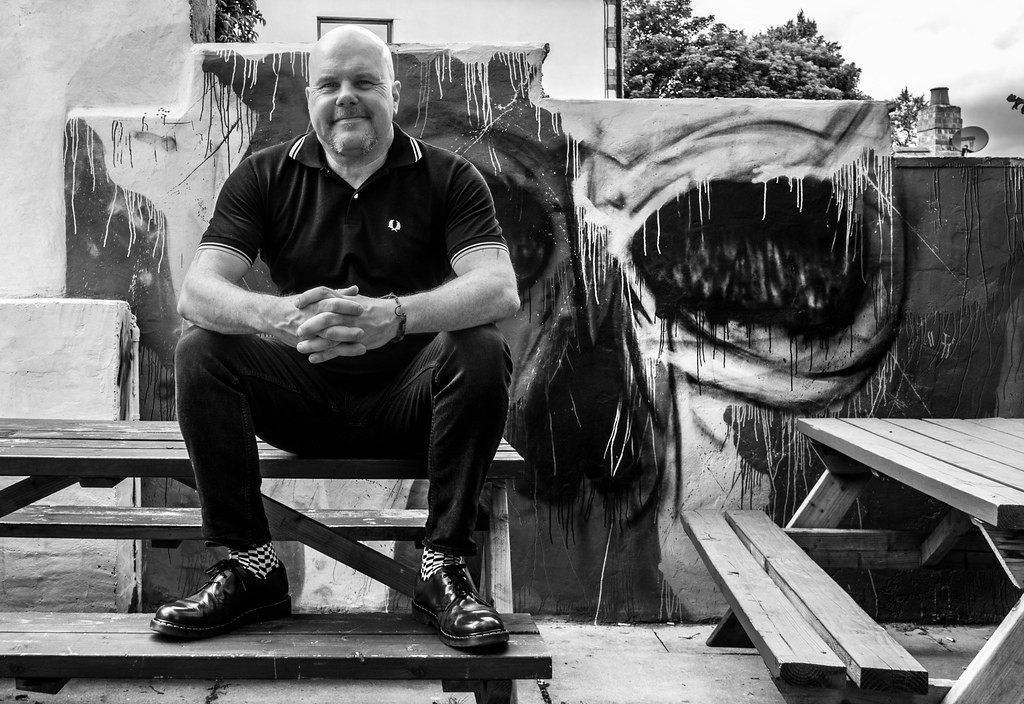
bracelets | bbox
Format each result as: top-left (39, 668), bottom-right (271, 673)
top-left (381, 292), bottom-right (407, 343)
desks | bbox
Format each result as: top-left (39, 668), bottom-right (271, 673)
top-left (0, 419), bottom-right (528, 704)
top-left (704, 417), bottom-right (1024, 704)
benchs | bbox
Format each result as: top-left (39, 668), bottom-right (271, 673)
top-left (0, 505), bottom-right (554, 704)
top-left (684, 508), bottom-right (928, 704)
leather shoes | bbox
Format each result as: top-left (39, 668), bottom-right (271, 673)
top-left (150, 559), bottom-right (291, 639)
top-left (412, 563), bottom-right (509, 649)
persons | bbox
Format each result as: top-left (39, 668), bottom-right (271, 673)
top-left (149, 24), bottom-right (521, 645)
